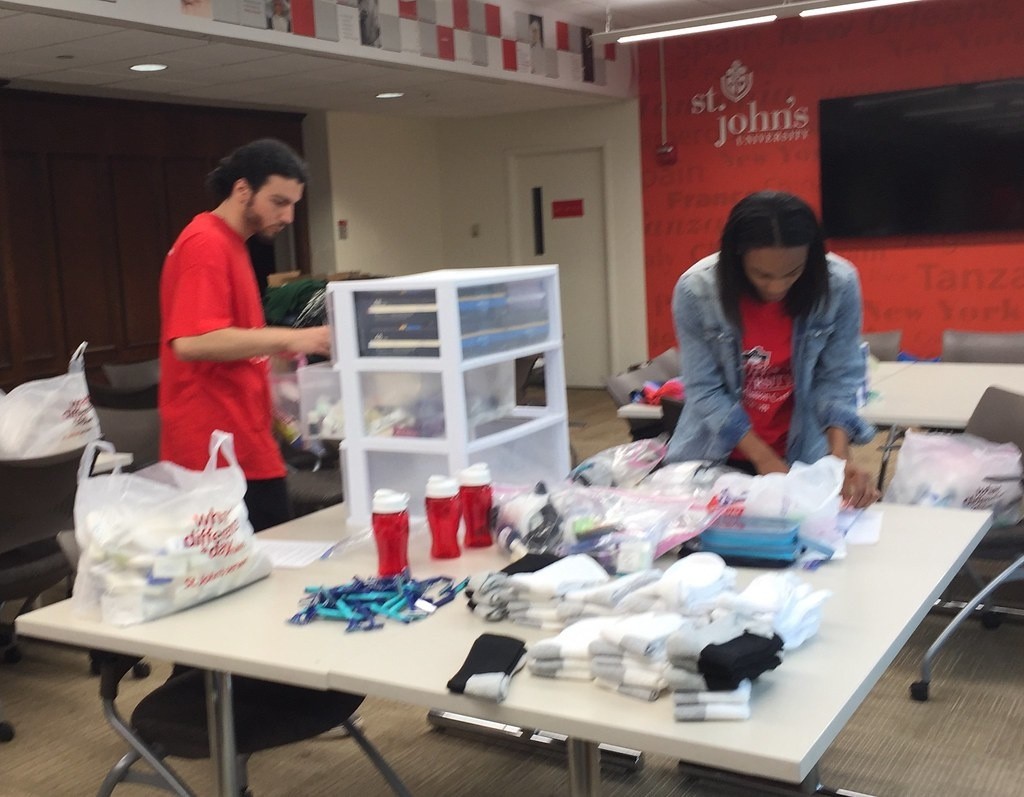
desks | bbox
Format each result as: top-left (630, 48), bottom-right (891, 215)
top-left (14, 482), bottom-right (995, 797)
top-left (618, 361), bottom-right (1024, 506)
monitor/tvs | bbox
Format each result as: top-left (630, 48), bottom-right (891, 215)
top-left (818, 77), bottom-right (1024, 239)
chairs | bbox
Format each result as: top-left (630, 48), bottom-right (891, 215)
top-left (864, 331), bottom-right (906, 361)
top-left (940, 329), bottom-right (1024, 363)
top-left (908, 384), bottom-right (1024, 701)
top-left (92, 650), bottom-right (410, 796)
top-left (602, 347), bottom-right (686, 406)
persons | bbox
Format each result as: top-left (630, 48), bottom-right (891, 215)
top-left (664, 188), bottom-right (881, 510)
top-left (158, 135), bottom-right (363, 530)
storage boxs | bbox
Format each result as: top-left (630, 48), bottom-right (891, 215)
top-left (293, 276), bottom-right (558, 517)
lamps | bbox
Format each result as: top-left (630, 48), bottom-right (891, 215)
top-left (586, 0), bottom-right (920, 48)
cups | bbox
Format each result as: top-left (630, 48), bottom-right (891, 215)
top-left (462, 463), bottom-right (493, 548)
top-left (426, 476), bottom-right (461, 559)
top-left (372, 489), bottom-right (410, 582)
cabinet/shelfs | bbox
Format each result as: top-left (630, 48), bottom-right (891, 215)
top-left (330, 262), bottom-right (573, 520)
